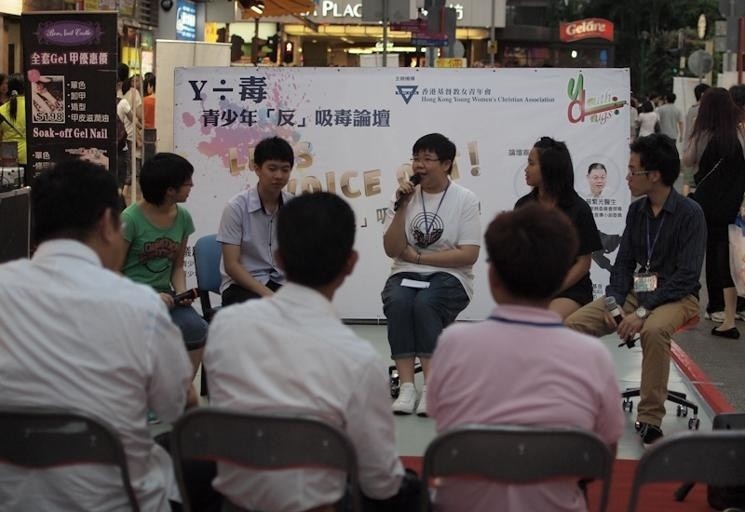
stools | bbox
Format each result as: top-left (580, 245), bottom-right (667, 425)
top-left (621, 316), bottom-right (701, 429)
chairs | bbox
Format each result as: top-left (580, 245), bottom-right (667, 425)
top-left (627, 431), bottom-right (744, 510)
top-left (191, 233), bottom-right (222, 394)
top-left (175, 408), bottom-right (363, 512)
top-left (418, 424), bottom-right (611, 510)
top-left (1, 407), bottom-right (144, 511)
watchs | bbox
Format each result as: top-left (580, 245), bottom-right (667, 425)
top-left (635, 307), bottom-right (649, 318)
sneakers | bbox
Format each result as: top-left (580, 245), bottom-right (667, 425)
top-left (639, 422), bottom-right (663, 448)
top-left (417, 385), bottom-right (428, 416)
top-left (705, 311), bottom-right (745, 338)
top-left (391, 382), bottom-right (417, 413)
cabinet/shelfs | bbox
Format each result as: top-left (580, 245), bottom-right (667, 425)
top-left (1, 186), bottom-right (37, 262)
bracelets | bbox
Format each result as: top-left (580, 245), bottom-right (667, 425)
top-left (414, 254), bottom-right (424, 264)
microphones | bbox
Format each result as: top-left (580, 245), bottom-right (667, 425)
top-left (173, 288), bottom-right (202, 304)
top-left (603, 296), bottom-right (636, 349)
top-left (394, 173), bottom-right (420, 211)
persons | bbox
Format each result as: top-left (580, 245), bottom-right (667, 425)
top-left (0, 158), bottom-right (200, 510)
top-left (421, 205), bottom-right (626, 508)
top-left (629, 82), bottom-right (743, 339)
top-left (584, 161), bottom-right (608, 197)
top-left (0, 65), bottom-right (155, 198)
top-left (118, 148), bottom-right (209, 346)
top-left (383, 131), bottom-right (481, 418)
top-left (566, 134), bottom-right (705, 443)
top-left (216, 133), bottom-right (300, 314)
top-left (204, 183), bottom-right (407, 510)
top-left (516, 137), bottom-right (596, 326)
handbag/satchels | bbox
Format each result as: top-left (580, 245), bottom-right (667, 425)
top-left (728, 214), bottom-right (745, 298)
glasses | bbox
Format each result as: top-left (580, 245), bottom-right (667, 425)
top-left (410, 157), bottom-right (441, 166)
